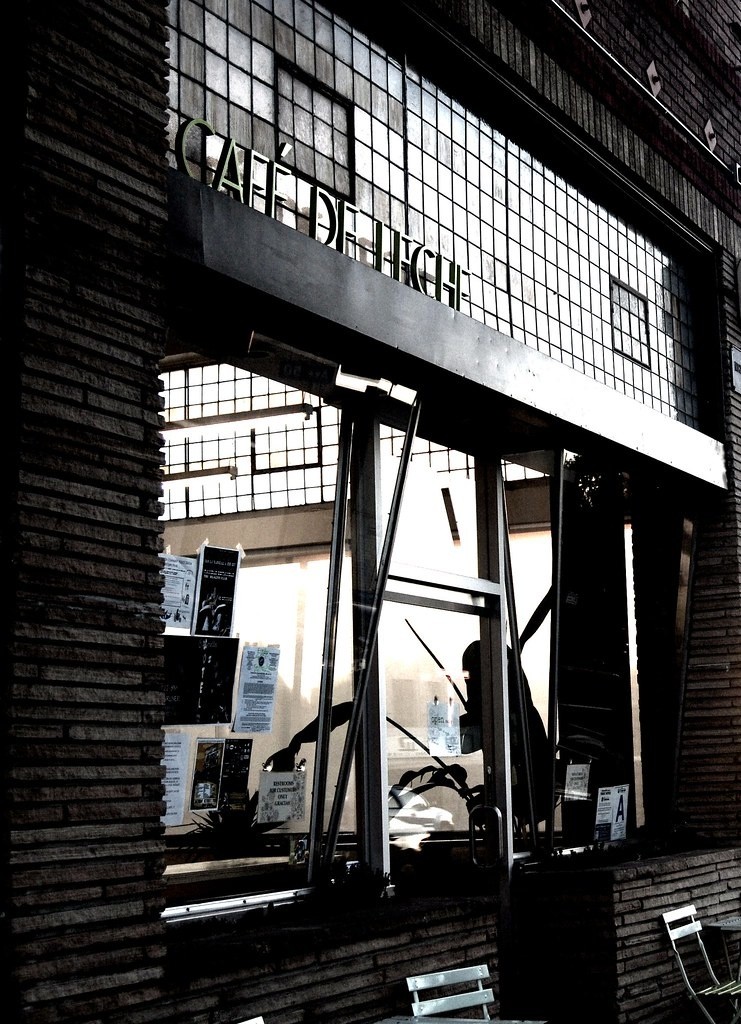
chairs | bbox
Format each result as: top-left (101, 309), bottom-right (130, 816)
top-left (405, 963), bottom-right (498, 1021)
top-left (661, 902), bottom-right (741, 1024)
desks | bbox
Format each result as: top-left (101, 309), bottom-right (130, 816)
top-left (702, 915), bottom-right (741, 1017)
top-left (370, 1015), bottom-right (548, 1024)
